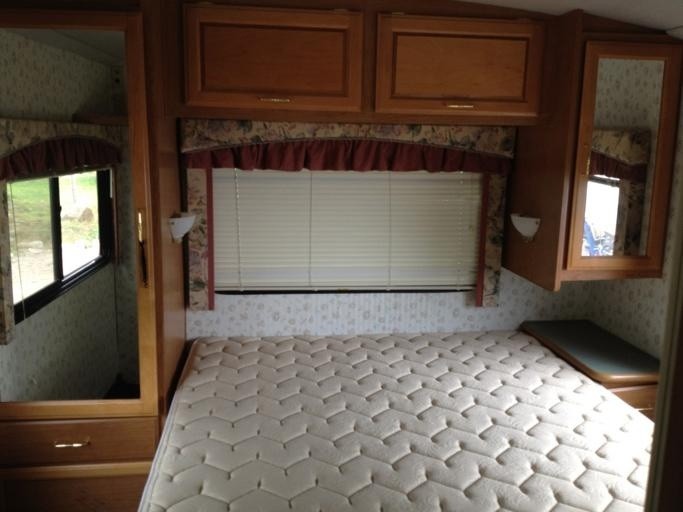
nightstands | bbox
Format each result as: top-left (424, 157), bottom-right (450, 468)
top-left (516, 318), bottom-right (662, 420)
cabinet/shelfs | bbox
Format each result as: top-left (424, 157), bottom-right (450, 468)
top-left (501, 9), bottom-right (683, 290)
top-left (1, 0), bottom-right (187, 512)
top-left (184, 3), bottom-right (545, 126)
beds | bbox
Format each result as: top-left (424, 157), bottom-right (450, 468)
top-left (135, 119), bottom-right (655, 511)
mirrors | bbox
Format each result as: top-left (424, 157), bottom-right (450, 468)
top-left (566, 39), bottom-right (683, 270)
top-left (0, 11), bottom-right (159, 421)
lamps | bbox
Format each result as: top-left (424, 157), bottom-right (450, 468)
top-left (168, 211), bottom-right (196, 244)
top-left (511, 214), bottom-right (541, 244)
